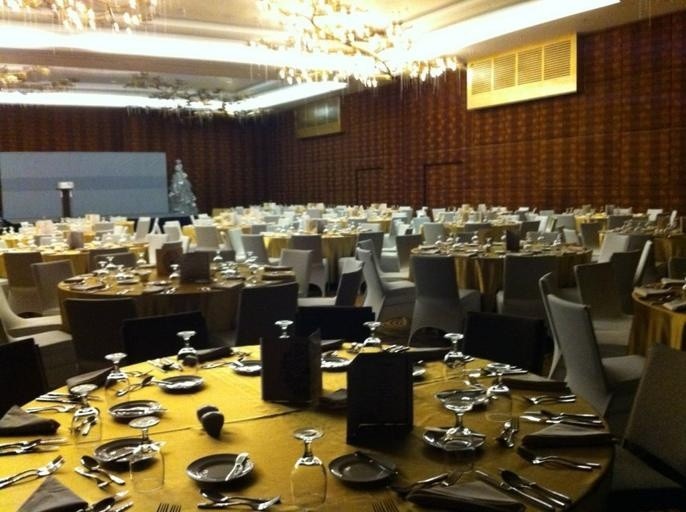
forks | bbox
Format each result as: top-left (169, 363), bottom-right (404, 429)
top-left (24, 403), bottom-right (77, 416)
top-left (519, 399), bottom-right (579, 406)
top-left (496, 461), bottom-right (571, 505)
top-left (193, 496), bottom-right (281, 510)
top-left (514, 449), bottom-right (603, 473)
top-left (0, 454), bottom-right (62, 482)
top-left (474, 466), bottom-right (566, 512)
top-left (519, 417), bottom-right (602, 429)
top-left (526, 392), bottom-right (575, 400)
top-left (72, 469), bottom-right (110, 491)
top-left (526, 405), bottom-right (601, 423)
top-left (0, 439), bottom-right (42, 455)
top-left (398, 471), bottom-right (461, 501)
top-left (1, 457), bottom-right (65, 487)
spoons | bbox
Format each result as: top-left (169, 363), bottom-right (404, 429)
top-left (199, 488), bottom-right (279, 502)
top-left (395, 471), bottom-right (447, 493)
top-left (79, 452), bottom-right (124, 488)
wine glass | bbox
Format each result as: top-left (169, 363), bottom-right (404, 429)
top-left (440, 333), bottom-right (466, 384)
top-left (125, 413), bottom-right (165, 494)
top-left (483, 360), bottom-right (513, 425)
top-left (174, 327), bottom-right (198, 372)
top-left (102, 349), bottom-right (127, 406)
top-left (361, 320), bottom-right (382, 354)
top-left (284, 422), bottom-right (330, 508)
top-left (65, 384), bottom-right (102, 444)
top-left (435, 379), bottom-right (474, 453)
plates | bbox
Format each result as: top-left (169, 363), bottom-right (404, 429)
top-left (108, 401), bottom-right (163, 422)
top-left (183, 450), bottom-right (257, 494)
top-left (422, 425), bottom-right (486, 452)
top-left (317, 352), bottom-right (351, 370)
top-left (157, 373), bottom-right (205, 395)
top-left (632, 275), bottom-right (686, 311)
top-left (325, 447), bottom-right (398, 487)
top-left (232, 356), bottom-right (261, 376)
top-left (259, 216), bottom-right (383, 235)
top-left (93, 435), bottom-right (151, 466)
top-left (62, 247), bottom-right (293, 294)
top-left (418, 231), bottom-right (586, 256)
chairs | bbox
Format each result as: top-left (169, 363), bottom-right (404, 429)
top-left (0, 201), bottom-right (686, 512)
